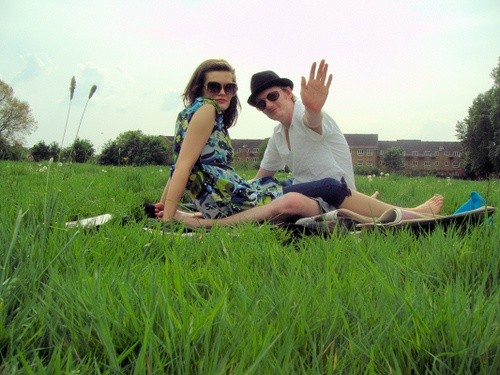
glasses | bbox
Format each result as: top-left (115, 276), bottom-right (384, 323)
top-left (203, 81), bottom-right (239, 97)
top-left (256, 89), bottom-right (284, 111)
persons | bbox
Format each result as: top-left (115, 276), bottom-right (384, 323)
top-left (140, 58), bottom-right (443, 224)
top-left (155, 59), bottom-right (361, 229)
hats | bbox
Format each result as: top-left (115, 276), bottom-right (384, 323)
top-left (247, 71), bottom-right (294, 108)
